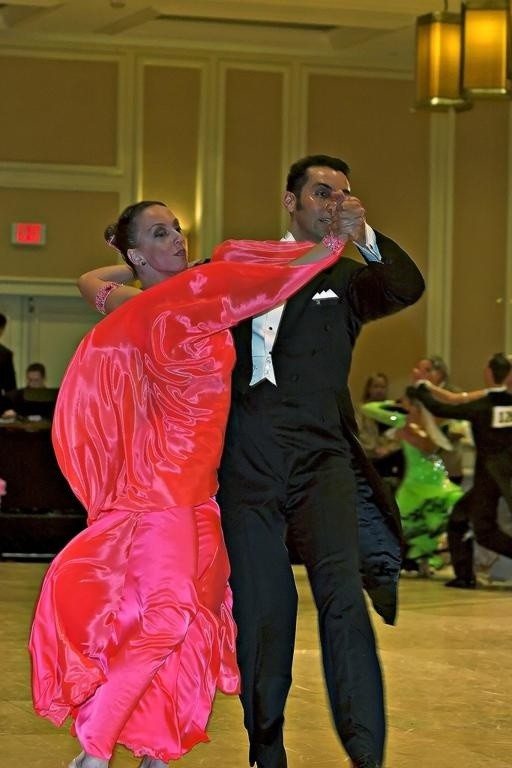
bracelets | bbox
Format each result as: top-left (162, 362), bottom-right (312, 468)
top-left (320, 232), bottom-right (346, 256)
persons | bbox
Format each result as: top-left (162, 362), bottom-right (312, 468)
top-left (22, 187), bottom-right (365, 767)
top-left (1, 311), bottom-right (19, 397)
top-left (349, 348), bottom-right (512, 598)
top-left (186, 154), bottom-right (429, 768)
top-left (0, 360), bottom-right (50, 422)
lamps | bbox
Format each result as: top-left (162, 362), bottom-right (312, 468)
top-left (413, 0), bottom-right (511, 112)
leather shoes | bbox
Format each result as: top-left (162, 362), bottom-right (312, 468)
top-left (444, 575), bottom-right (477, 591)
top-left (135, 754), bottom-right (154, 768)
top-left (65, 750), bottom-right (86, 768)
